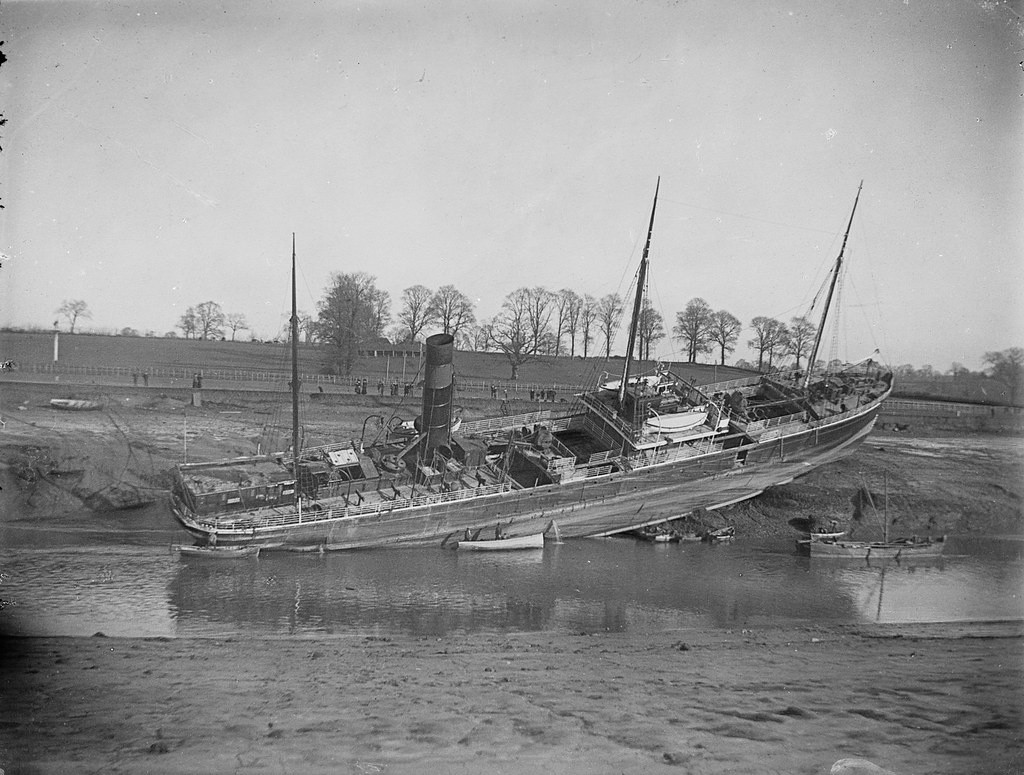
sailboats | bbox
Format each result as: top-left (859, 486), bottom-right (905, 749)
top-left (166, 174), bottom-right (897, 554)
top-left (794, 468), bottom-right (949, 561)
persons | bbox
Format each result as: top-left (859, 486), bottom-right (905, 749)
top-left (530, 389), bottom-right (535, 401)
top-left (404, 383), bottom-right (414, 397)
top-left (193, 373), bottom-right (203, 388)
top-left (491, 384), bottom-right (499, 397)
top-left (143, 372), bottom-right (148, 386)
top-left (504, 388), bottom-right (508, 398)
top-left (355, 380), bottom-right (367, 394)
top-left (318, 386), bottom-right (323, 392)
top-left (377, 381), bottom-right (384, 396)
top-left (390, 382), bottom-right (398, 395)
top-left (541, 389), bottom-right (557, 400)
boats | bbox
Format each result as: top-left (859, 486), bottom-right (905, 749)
top-left (171, 542), bottom-right (260, 559)
top-left (50, 398), bottom-right (106, 412)
top-left (456, 532), bottom-right (544, 555)
